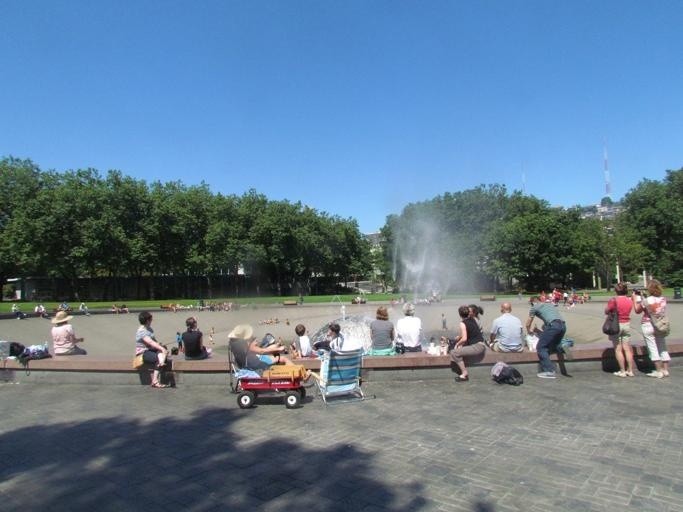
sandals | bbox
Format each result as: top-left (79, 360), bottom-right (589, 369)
top-left (150, 381), bottom-right (169, 388)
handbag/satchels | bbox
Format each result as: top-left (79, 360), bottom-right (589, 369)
top-left (653, 314), bottom-right (669, 338)
top-left (131, 353), bottom-right (144, 370)
top-left (601, 318), bottom-right (619, 334)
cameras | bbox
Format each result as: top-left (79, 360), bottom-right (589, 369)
top-left (633, 288), bottom-right (641, 296)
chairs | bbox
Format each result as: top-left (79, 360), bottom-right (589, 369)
top-left (312, 343), bottom-right (368, 405)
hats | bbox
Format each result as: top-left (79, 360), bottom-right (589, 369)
top-left (226, 324), bottom-right (253, 340)
top-left (49, 311), bottom-right (73, 325)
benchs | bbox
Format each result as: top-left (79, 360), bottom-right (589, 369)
top-left (0, 304), bottom-right (215, 321)
top-left (0, 338), bottom-right (682, 385)
top-left (529, 295), bottom-right (611, 303)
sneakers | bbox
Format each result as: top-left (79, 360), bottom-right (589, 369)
top-left (536, 370), bottom-right (557, 379)
top-left (613, 368), bottom-right (670, 378)
top-left (454, 374), bottom-right (468, 381)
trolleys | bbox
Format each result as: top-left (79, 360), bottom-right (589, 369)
top-left (226, 344), bottom-right (312, 409)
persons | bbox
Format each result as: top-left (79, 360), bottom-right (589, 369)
top-left (604, 283), bottom-right (635, 377)
top-left (540, 287), bottom-right (589, 306)
top-left (526, 296), bottom-right (567, 378)
top-left (390, 288), bottom-right (441, 305)
top-left (441, 314), bottom-right (448, 332)
top-left (370, 303), bottom-right (422, 353)
top-left (50, 310), bottom-right (88, 356)
top-left (228, 318), bottom-right (344, 381)
top-left (112, 304), bottom-right (129, 315)
top-left (352, 296), bottom-right (366, 304)
top-left (632, 279), bottom-right (671, 378)
top-left (176, 317), bottom-right (215, 360)
top-left (12, 304), bottom-right (25, 320)
top-left (79, 303), bottom-right (90, 316)
top-left (486, 301), bottom-right (525, 352)
top-left (451, 304), bottom-right (485, 382)
top-left (35, 301), bottom-right (72, 319)
top-left (135, 312), bottom-right (171, 388)
top-left (198, 299), bottom-right (233, 312)
